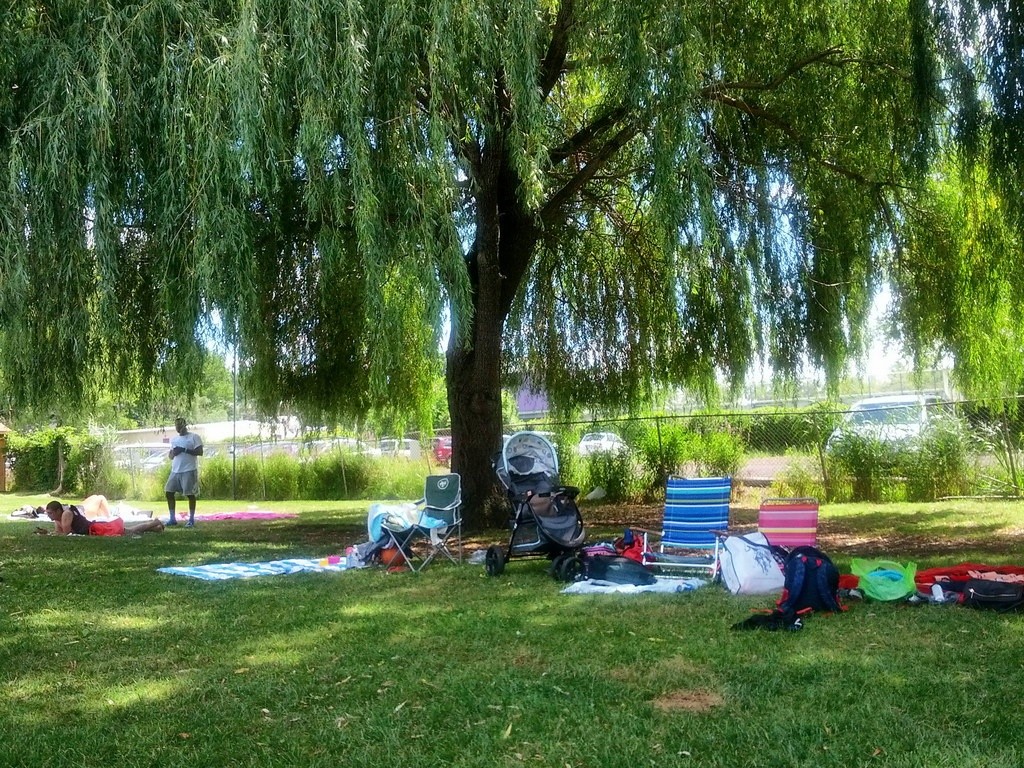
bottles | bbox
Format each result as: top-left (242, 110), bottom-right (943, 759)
top-left (351, 544), bottom-right (359, 566)
top-left (346, 547), bottom-right (352, 568)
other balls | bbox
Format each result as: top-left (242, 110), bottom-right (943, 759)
top-left (381, 549), bottom-right (405, 567)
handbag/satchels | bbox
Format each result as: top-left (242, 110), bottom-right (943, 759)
top-left (617, 528), bottom-right (656, 569)
top-left (718, 531), bottom-right (785, 594)
top-left (588, 554), bottom-right (656, 587)
top-left (964, 579), bottom-right (1024, 614)
top-left (580, 542), bottom-right (615, 571)
top-left (850, 557), bottom-right (918, 603)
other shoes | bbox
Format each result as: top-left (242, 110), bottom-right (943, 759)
top-left (184, 521), bottom-right (193, 527)
top-left (164, 521), bottom-right (178, 526)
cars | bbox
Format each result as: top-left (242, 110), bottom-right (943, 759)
top-left (503, 431), bottom-right (579, 457)
top-left (579, 432), bottom-right (629, 457)
top-left (826, 395), bottom-right (959, 453)
top-left (102, 436), bottom-right (452, 473)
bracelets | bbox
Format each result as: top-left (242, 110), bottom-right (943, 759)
top-left (184, 447), bottom-right (187, 453)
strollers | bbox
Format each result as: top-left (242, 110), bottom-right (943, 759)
top-left (485, 431), bottom-right (587, 584)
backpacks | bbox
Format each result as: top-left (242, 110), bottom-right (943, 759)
top-left (782, 545), bottom-right (843, 615)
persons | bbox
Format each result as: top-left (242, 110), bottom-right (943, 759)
top-left (36, 495), bottom-right (164, 536)
top-left (164, 418), bottom-right (203, 527)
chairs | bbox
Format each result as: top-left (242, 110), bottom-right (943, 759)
top-left (380, 471), bottom-right (463, 572)
top-left (628, 474), bottom-right (732, 582)
top-left (758, 496), bottom-right (818, 546)
